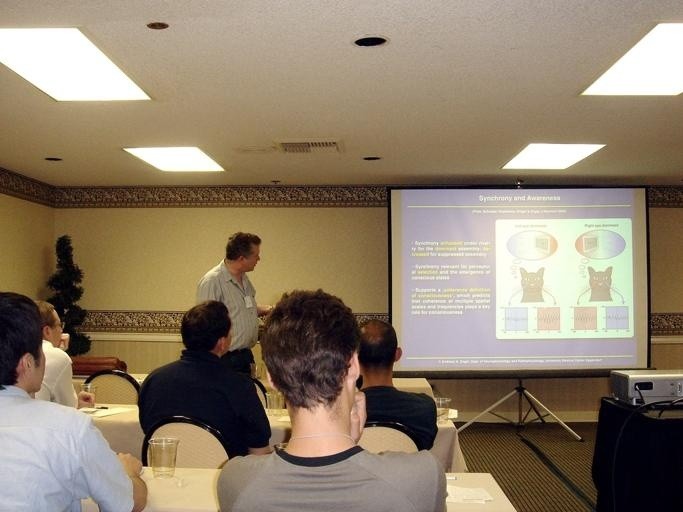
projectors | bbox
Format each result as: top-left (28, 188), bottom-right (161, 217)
top-left (610, 369), bottom-right (683, 405)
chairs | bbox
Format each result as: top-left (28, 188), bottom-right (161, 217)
top-left (253, 377), bottom-right (270, 411)
top-left (142, 415), bottom-right (235, 467)
top-left (84, 369), bottom-right (142, 404)
top-left (356, 420), bottom-right (421, 455)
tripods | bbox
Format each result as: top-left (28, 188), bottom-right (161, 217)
top-left (457, 380), bottom-right (583, 443)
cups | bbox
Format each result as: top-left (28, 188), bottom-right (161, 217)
top-left (147, 438), bottom-right (179, 477)
top-left (77, 382), bottom-right (95, 410)
top-left (56, 333), bottom-right (69, 351)
top-left (264, 391), bottom-right (282, 416)
top-left (434, 397), bottom-right (450, 423)
top-left (249, 363), bottom-right (261, 378)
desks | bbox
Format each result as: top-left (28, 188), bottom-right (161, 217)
top-left (69, 371), bottom-right (438, 405)
top-left (80, 406), bottom-right (470, 473)
top-left (80, 468), bottom-right (518, 512)
top-left (589, 397), bottom-right (683, 512)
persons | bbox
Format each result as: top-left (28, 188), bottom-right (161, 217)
top-left (196, 231), bottom-right (275, 377)
top-left (216, 289), bottom-right (448, 511)
top-left (1, 293), bottom-right (148, 511)
top-left (138, 300), bottom-right (272, 467)
top-left (29, 299), bottom-right (96, 414)
top-left (357, 317), bottom-right (437, 453)
